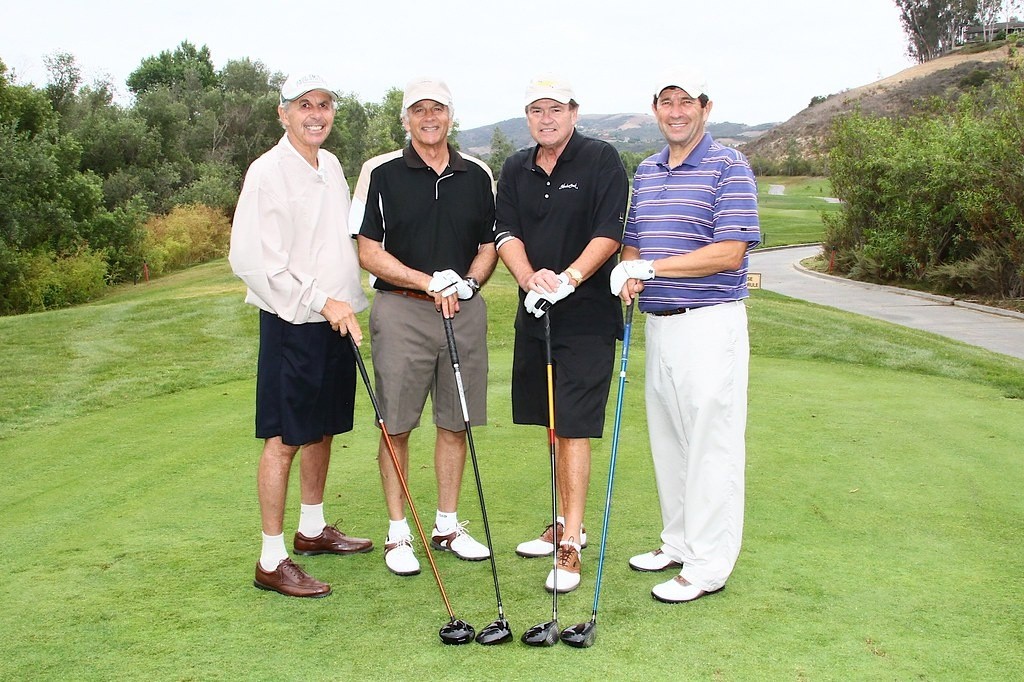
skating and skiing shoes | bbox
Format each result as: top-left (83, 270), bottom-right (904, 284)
top-left (544, 536), bottom-right (581, 593)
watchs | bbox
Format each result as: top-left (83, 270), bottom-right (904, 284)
top-left (462, 277), bottom-right (480, 294)
top-left (565, 267), bottom-right (583, 287)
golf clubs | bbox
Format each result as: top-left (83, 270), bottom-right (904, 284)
top-left (521, 310), bottom-right (561, 649)
top-left (345, 331), bottom-right (475, 645)
top-left (441, 306), bottom-right (514, 647)
top-left (562, 296), bottom-right (634, 648)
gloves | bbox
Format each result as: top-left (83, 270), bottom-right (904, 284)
top-left (428, 269), bottom-right (473, 299)
top-left (609, 259), bottom-right (654, 295)
top-left (524, 272), bottom-right (575, 318)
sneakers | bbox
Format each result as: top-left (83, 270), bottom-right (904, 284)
top-left (516, 522), bottom-right (588, 556)
top-left (652, 574), bottom-right (726, 602)
top-left (630, 548), bottom-right (683, 572)
top-left (383, 535), bottom-right (421, 575)
top-left (430, 522), bottom-right (490, 561)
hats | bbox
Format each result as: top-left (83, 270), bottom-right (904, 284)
top-left (403, 80), bottom-right (454, 109)
top-left (653, 66), bottom-right (710, 102)
top-left (525, 75), bottom-right (581, 106)
top-left (280, 70), bottom-right (339, 106)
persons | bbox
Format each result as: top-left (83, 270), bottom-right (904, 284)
top-left (230, 72), bottom-right (374, 599)
top-left (348, 74), bottom-right (499, 578)
top-left (609, 67), bottom-right (762, 605)
top-left (493, 68), bottom-right (630, 596)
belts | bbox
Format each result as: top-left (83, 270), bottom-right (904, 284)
top-left (392, 290), bottom-right (435, 303)
top-left (647, 299), bottom-right (742, 316)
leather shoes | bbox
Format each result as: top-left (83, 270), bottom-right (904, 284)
top-left (293, 519), bottom-right (374, 556)
top-left (254, 557), bottom-right (332, 598)
